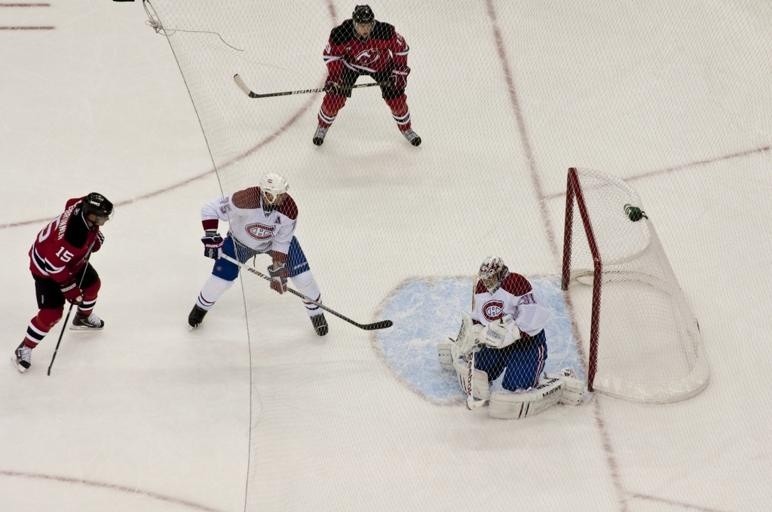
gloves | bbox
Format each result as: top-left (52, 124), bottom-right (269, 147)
top-left (200, 234), bottom-right (225, 261)
top-left (60, 283), bottom-right (85, 306)
top-left (388, 64), bottom-right (410, 95)
top-left (325, 76), bottom-right (352, 98)
top-left (267, 261), bottom-right (289, 295)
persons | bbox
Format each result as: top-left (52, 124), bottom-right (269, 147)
top-left (185, 172), bottom-right (329, 337)
top-left (10, 191), bottom-right (114, 374)
top-left (312, 4), bottom-right (422, 146)
top-left (451, 256), bottom-right (585, 420)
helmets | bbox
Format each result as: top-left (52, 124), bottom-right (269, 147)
top-left (82, 192), bottom-right (115, 217)
top-left (476, 255), bottom-right (508, 294)
top-left (258, 173), bottom-right (290, 206)
top-left (352, 5), bottom-right (375, 33)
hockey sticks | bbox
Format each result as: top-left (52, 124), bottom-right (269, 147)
top-left (466, 275), bottom-right (489, 410)
top-left (221, 252), bottom-right (393, 330)
top-left (47, 252), bottom-right (91, 375)
top-left (233, 73), bottom-right (390, 98)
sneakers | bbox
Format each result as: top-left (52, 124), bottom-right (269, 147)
top-left (311, 313), bottom-right (328, 335)
top-left (188, 304), bottom-right (207, 326)
top-left (313, 124), bottom-right (329, 145)
top-left (72, 313), bottom-right (105, 328)
top-left (400, 127), bottom-right (421, 147)
top-left (15, 342), bottom-right (32, 369)
